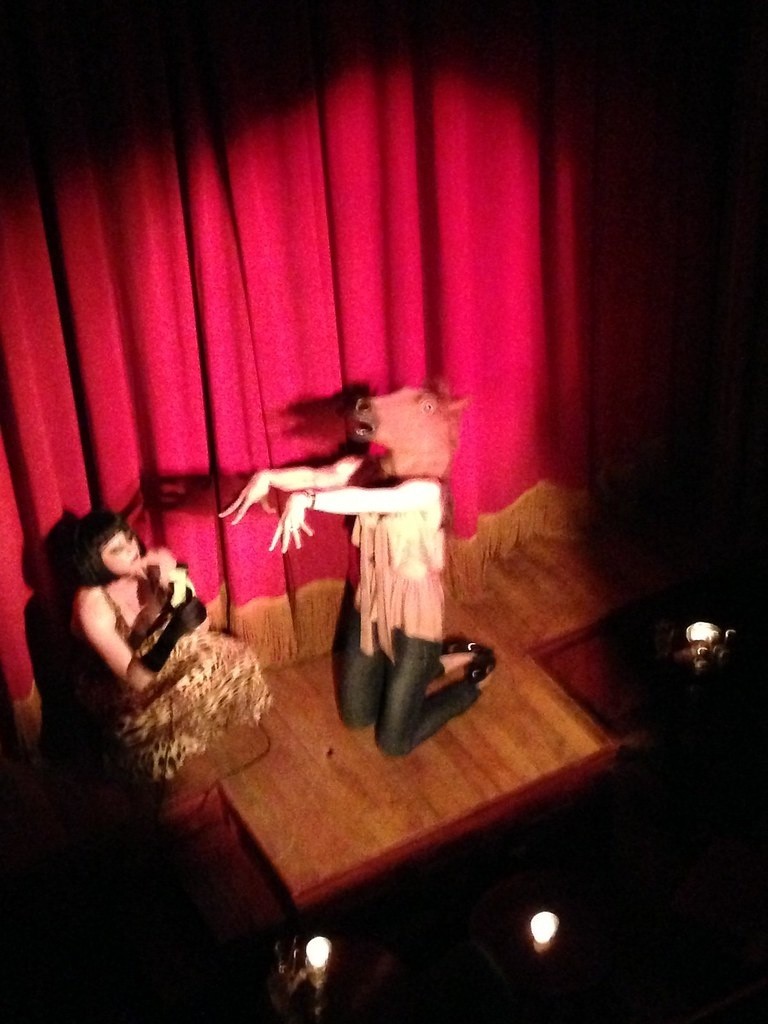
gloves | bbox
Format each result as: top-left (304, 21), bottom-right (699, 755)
top-left (141, 597), bottom-right (207, 672)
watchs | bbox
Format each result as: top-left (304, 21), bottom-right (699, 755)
top-left (304, 489), bottom-right (315, 512)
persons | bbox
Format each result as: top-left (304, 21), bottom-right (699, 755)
top-left (218, 387), bottom-right (495, 756)
top-left (62, 508), bottom-right (274, 789)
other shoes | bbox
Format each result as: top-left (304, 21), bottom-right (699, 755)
top-left (439, 640), bottom-right (480, 655)
top-left (467, 654), bottom-right (496, 685)
